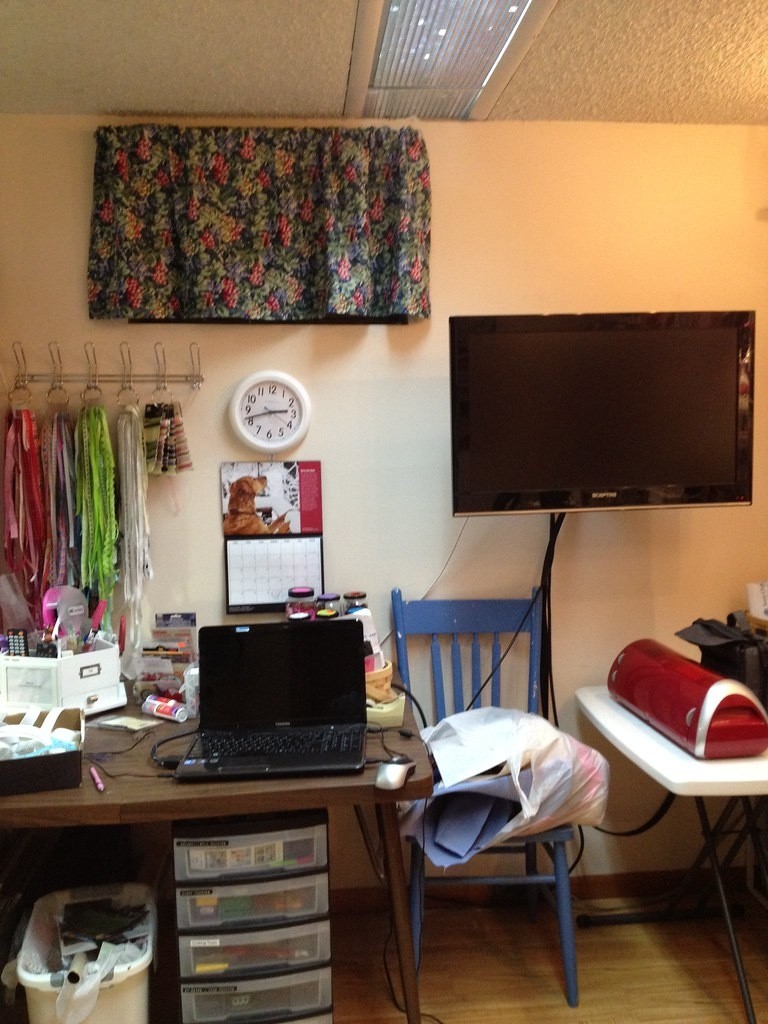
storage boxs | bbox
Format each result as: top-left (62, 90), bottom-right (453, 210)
top-left (0, 638), bottom-right (128, 719)
top-left (176, 963), bottom-right (335, 1024)
top-left (174, 914), bottom-right (334, 983)
top-left (170, 805), bottom-right (330, 888)
top-left (173, 869), bottom-right (331, 934)
top-left (365, 661), bottom-right (407, 731)
top-left (269, 1008), bottom-right (334, 1024)
top-left (0, 707), bottom-right (87, 799)
top-left (17, 880), bottom-right (155, 1024)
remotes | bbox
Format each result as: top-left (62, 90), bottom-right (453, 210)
top-left (36, 643), bottom-right (56, 658)
top-left (7, 629), bottom-right (27, 657)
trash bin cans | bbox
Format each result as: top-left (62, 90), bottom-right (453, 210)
top-left (16, 882), bottom-right (156, 1024)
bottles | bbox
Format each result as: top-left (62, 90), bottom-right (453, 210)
top-left (284, 586), bottom-right (368, 621)
top-left (141, 694), bottom-right (189, 723)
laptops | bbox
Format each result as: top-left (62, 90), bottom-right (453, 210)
top-left (175, 619), bottom-right (366, 780)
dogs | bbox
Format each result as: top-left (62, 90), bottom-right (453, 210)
top-left (222, 475), bottom-right (290, 534)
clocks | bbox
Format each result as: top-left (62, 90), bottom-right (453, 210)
top-left (227, 371), bottom-right (312, 456)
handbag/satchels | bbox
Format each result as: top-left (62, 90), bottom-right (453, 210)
top-left (675, 608), bottom-right (768, 712)
top-left (399, 700), bottom-right (609, 852)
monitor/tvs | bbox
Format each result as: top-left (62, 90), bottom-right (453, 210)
top-left (450, 311), bottom-right (753, 515)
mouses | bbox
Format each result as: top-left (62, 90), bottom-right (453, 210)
top-left (374, 757), bottom-right (416, 790)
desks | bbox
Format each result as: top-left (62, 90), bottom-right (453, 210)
top-left (576, 685), bottom-right (768, 1024)
top-left (0, 678), bottom-right (434, 1024)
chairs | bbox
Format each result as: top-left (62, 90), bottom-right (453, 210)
top-left (391, 586), bottom-right (583, 1010)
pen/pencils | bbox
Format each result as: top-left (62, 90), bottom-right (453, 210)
top-left (89, 766), bottom-right (105, 791)
